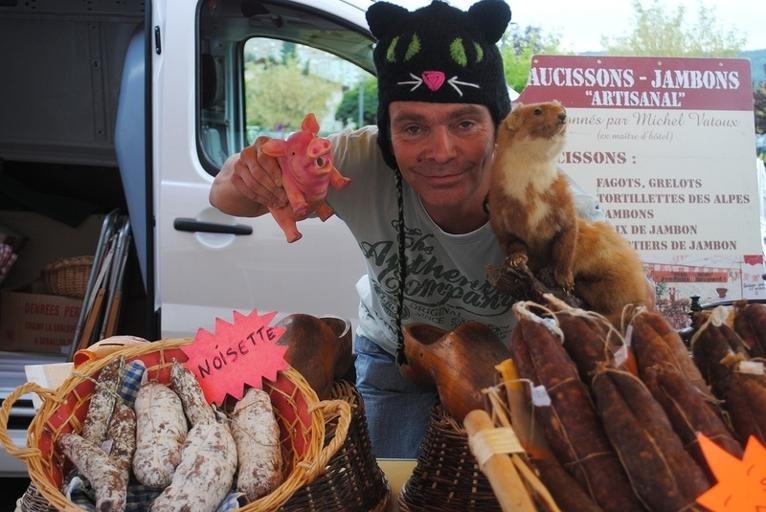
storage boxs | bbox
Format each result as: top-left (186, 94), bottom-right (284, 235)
top-left (0, 283), bottom-right (83, 356)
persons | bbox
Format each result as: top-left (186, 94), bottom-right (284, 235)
top-left (207, 0), bottom-right (606, 460)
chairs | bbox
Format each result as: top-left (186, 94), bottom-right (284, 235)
top-left (198, 54), bottom-right (227, 166)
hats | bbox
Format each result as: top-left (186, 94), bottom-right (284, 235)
top-left (366, 0), bottom-right (511, 170)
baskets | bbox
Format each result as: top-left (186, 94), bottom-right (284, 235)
top-left (398, 402), bottom-right (503, 511)
top-left (43, 256), bottom-right (93, 298)
top-left (0, 338), bottom-right (390, 512)
top-left (0, 338), bottom-right (390, 511)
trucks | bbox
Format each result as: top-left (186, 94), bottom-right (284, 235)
top-left (0, 0), bottom-right (766, 483)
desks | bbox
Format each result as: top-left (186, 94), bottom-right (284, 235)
top-left (370, 458), bottom-right (421, 509)
